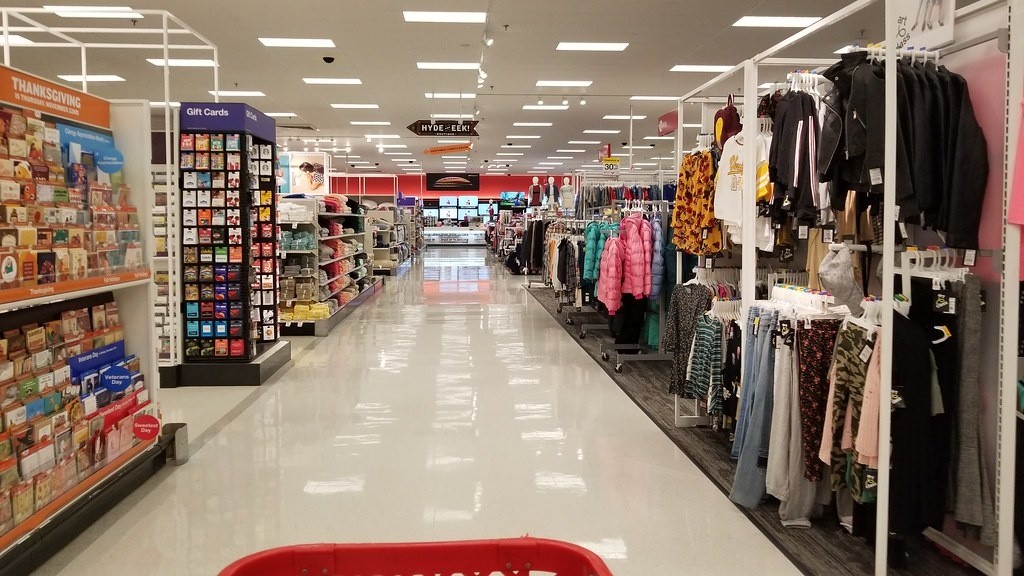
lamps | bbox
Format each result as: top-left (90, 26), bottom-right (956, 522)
top-left (562, 96), bottom-right (569, 105)
top-left (579, 95), bottom-right (586, 105)
top-left (477, 75), bottom-right (484, 83)
top-left (482, 33), bottom-right (494, 47)
top-left (478, 68), bottom-right (488, 78)
top-left (538, 95), bottom-right (545, 106)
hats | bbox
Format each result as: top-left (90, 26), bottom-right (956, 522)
top-left (816, 242), bottom-right (865, 318)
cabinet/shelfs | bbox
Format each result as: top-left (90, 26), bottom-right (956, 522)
top-left (0, 100), bottom-right (431, 550)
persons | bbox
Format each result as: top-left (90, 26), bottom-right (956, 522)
top-left (560, 177), bottom-right (575, 210)
top-left (299, 162), bottom-right (325, 190)
top-left (486, 199), bottom-right (494, 221)
top-left (528, 176), bottom-right (543, 207)
top-left (545, 177), bottom-right (559, 203)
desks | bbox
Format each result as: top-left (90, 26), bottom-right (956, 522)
top-left (422, 227), bottom-right (487, 245)
